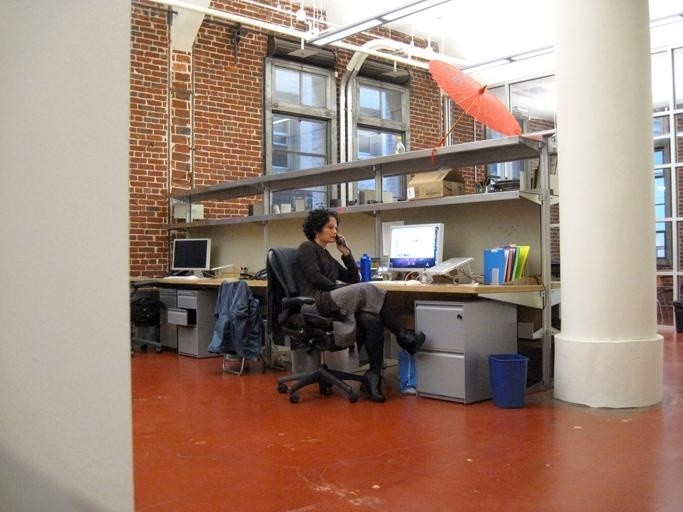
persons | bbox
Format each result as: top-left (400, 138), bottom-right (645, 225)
top-left (291, 208), bottom-right (425, 403)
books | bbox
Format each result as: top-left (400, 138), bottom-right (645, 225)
top-left (364, 279), bottom-right (422, 286)
top-left (482, 244), bottom-right (532, 285)
top-left (162, 275), bottom-right (199, 281)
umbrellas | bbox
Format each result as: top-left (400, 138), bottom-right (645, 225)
top-left (425, 59), bottom-right (522, 148)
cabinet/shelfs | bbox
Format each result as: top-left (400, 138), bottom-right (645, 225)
top-left (415, 301), bottom-right (520, 406)
top-left (173, 137), bottom-right (559, 282)
top-left (167, 287), bottom-right (224, 359)
top-left (155, 286), bottom-right (179, 353)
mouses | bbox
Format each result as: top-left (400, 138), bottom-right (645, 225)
top-left (404, 280), bottom-right (421, 286)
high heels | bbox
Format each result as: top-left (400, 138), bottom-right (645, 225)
top-left (360, 369), bottom-right (385, 403)
top-left (396, 328), bottom-right (425, 355)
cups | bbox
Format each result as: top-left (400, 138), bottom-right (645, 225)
top-left (382, 262), bottom-right (393, 280)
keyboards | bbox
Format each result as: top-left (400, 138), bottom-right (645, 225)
top-left (366, 280), bottom-right (405, 286)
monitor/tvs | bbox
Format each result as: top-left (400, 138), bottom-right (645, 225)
top-left (172, 238), bottom-right (211, 279)
top-left (387, 223), bottom-right (445, 281)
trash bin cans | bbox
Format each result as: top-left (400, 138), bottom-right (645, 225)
top-left (489, 354), bottom-right (529, 407)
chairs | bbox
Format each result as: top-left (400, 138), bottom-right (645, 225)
top-left (206, 279), bottom-right (287, 375)
top-left (262, 244), bottom-right (382, 403)
top-left (655, 275), bottom-right (672, 323)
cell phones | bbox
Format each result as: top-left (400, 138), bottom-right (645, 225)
top-left (335, 234), bottom-right (343, 247)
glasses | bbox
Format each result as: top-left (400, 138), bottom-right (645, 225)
top-left (255, 269), bottom-right (267, 280)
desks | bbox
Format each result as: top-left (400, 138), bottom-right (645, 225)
top-left (147, 274), bottom-right (560, 397)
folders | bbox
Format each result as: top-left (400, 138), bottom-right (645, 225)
top-left (484, 244), bottom-right (531, 283)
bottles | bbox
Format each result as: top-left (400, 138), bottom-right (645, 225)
top-left (360, 252), bottom-right (373, 281)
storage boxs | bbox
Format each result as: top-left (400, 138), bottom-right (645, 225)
top-left (407, 167), bottom-right (465, 202)
